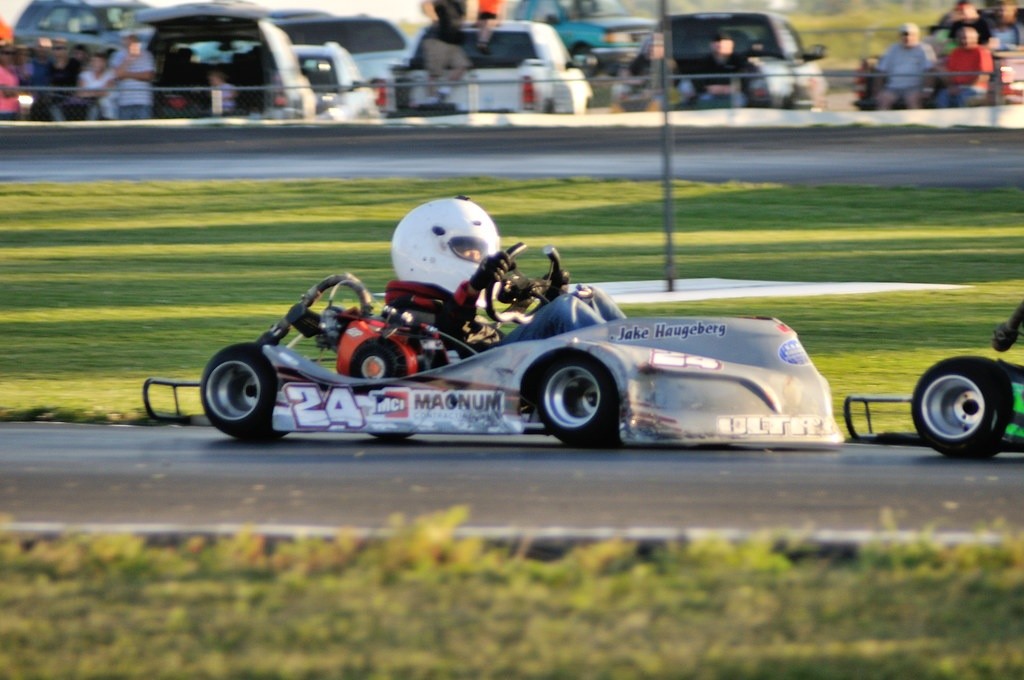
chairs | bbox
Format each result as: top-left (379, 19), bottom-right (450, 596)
top-left (725, 30), bottom-right (750, 54)
top-left (162, 48), bottom-right (265, 86)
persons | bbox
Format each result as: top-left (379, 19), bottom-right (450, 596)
top-left (420, 0), bottom-right (500, 110)
top-left (383, 194), bottom-right (629, 361)
top-left (621, 32), bottom-right (759, 110)
top-left (872, 0), bottom-right (1024, 111)
top-left (0, 19), bottom-right (194, 122)
top-left (206, 70), bottom-right (237, 115)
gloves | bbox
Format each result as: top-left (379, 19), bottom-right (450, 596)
top-left (469, 251), bottom-right (516, 291)
top-left (544, 270), bottom-right (569, 300)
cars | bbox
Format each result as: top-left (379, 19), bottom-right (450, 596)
top-left (10, 0), bottom-right (830, 113)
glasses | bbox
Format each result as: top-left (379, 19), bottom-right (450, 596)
top-left (3, 51), bottom-right (15, 56)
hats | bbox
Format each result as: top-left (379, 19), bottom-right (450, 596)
top-left (900, 22), bottom-right (918, 36)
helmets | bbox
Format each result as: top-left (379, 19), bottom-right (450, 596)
top-left (392, 198), bottom-right (500, 308)
